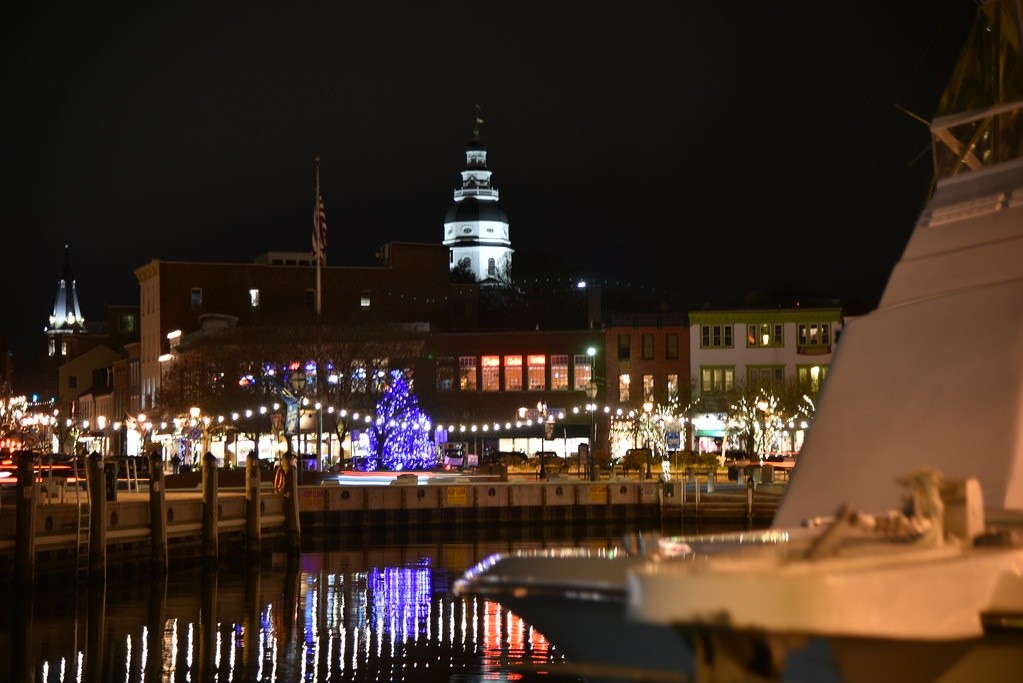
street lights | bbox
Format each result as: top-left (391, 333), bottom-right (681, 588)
top-left (586, 346), bottom-right (597, 482)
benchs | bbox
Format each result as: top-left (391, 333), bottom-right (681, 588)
top-left (683, 467), bottom-right (718, 481)
top-left (598, 466), bottom-right (629, 477)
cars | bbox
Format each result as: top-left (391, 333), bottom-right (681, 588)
top-left (443, 448), bottom-right (557, 474)
